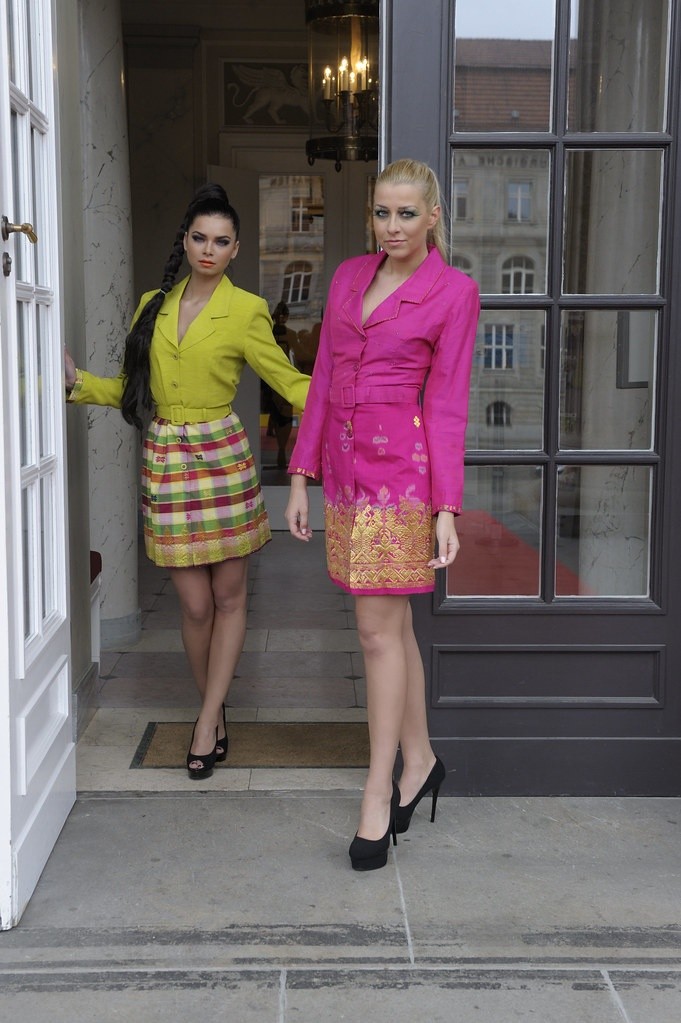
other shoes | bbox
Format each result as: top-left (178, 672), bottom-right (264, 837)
top-left (186, 718), bottom-right (219, 778)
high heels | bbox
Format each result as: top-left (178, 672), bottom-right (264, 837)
top-left (213, 700), bottom-right (228, 761)
top-left (349, 781), bottom-right (401, 871)
top-left (389, 755), bottom-right (446, 833)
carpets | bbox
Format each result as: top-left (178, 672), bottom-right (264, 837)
top-left (260, 425), bottom-right (299, 449)
top-left (446, 509), bottom-right (579, 596)
top-left (129, 721), bottom-right (370, 769)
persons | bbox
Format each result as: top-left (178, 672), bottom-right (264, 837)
top-left (65, 185), bottom-right (313, 781)
top-left (284, 160), bottom-right (479, 871)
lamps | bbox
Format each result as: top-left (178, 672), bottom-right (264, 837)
top-left (305, 0), bottom-right (379, 172)
